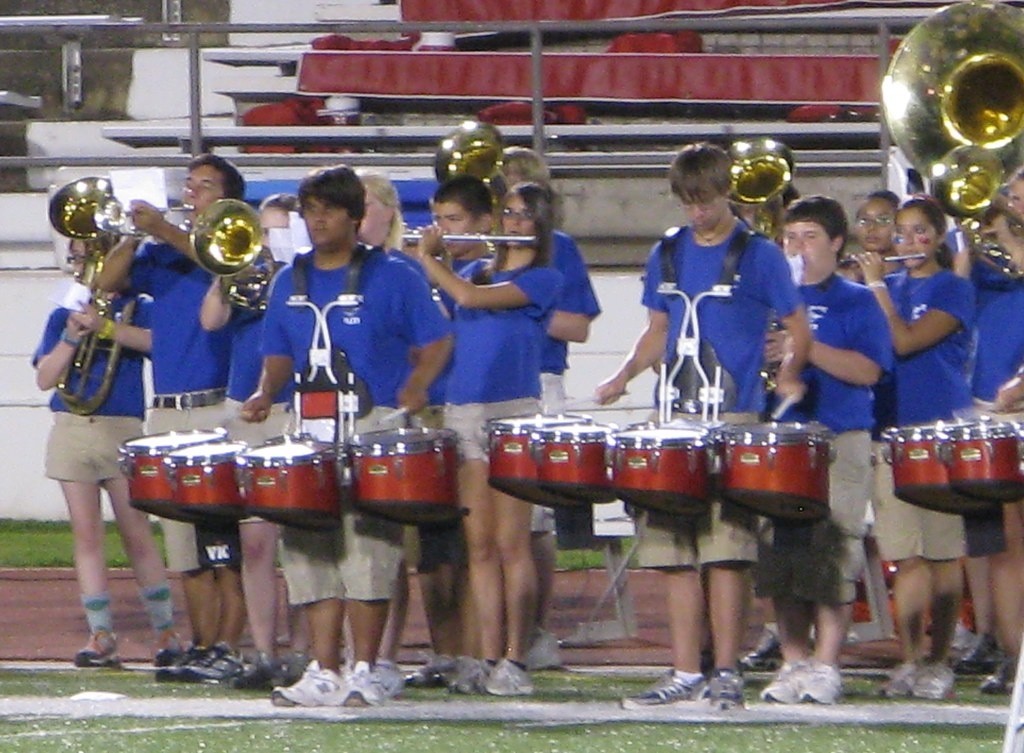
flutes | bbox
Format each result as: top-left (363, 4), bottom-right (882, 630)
top-left (835, 252), bottom-right (928, 269)
top-left (400, 234), bottom-right (541, 242)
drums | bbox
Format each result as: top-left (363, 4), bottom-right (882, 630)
top-left (611, 423), bottom-right (711, 517)
top-left (351, 427), bottom-right (459, 528)
top-left (718, 420), bottom-right (833, 524)
top-left (532, 421), bottom-right (622, 504)
top-left (947, 419), bottom-right (1024, 502)
top-left (166, 440), bottom-right (248, 523)
top-left (122, 427), bottom-right (228, 520)
top-left (240, 433), bottom-right (346, 529)
top-left (484, 414), bottom-right (590, 509)
top-left (892, 417), bottom-right (995, 521)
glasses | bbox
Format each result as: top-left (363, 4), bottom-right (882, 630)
top-left (858, 217), bottom-right (891, 226)
top-left (503, 208), bottom-right (532, 218)
top-left (677, 193), bottom-right (725, 210)
top-left (67, 256), bottom-right (87, 264)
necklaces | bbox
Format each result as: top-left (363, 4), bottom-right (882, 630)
top-left (692, 214), bottom-right (733, 244)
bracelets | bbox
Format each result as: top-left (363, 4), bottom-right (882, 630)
top-left (97, 317), bottom-right (115, 339)
top-left (868, 280), bottom-right (888, 290)
top-left (60, 328), bottom-right (82, 348)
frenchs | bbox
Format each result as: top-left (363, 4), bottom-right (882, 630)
top-left (882, 1), bottom-right (1024, 181)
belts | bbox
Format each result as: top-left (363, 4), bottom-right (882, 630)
top-left (153, 390), bottom-right (225, 408)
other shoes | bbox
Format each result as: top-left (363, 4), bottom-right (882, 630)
top-left (744, 635), bottom-right (784, 669)
top-left (980, 663), bottom-right (1008, 692)
top-left (340, 661), bottom-right (405, 697)
top-left (449, 660), bottom-right (534, 696)
top-left (405, 665), bottom-right (448, 687)
top-left (523, 628), bottom-right (563, 669)
top-left (955, 633), bottom-right (996, 674)
top-left (879, 664), bottom-right (953, 698)
top-left (233, 651), bottom-right (309, 689)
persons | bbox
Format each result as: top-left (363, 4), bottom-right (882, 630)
top-left (662, 163), bottom-right (1024, 706)
top-left (591, 141), bottom-right (812, 710)
top-left (31, 146), bottom-right (602, 708)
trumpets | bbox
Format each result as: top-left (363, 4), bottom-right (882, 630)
top-left (102, 195), bottom-right (197, 228)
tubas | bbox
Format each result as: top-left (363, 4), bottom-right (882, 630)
top-left (435, 120), bottom-right (514, 253)
top-left (48, 174), bottom-right (137, 418)
top-left (721, 137), bottom-right (796, 387)
top-left (190, 197), bottom-right (278, 314)
top-left (928, 146), bottom-right (1023, 284)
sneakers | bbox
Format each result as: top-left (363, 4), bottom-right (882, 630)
top-left (155, 645), bottom-right (243, 683)
top-left (271, 660), bottom-right (385, 706)
top-left (152, 628), bottom-right (184, 666)
top-left (74, 629), bottom-right (120, 666)
top-left (759, 661), bottom-right (841, 705)
top-left (622, 668), bottom-right (712, 710)
top-left (711, 673), bottom-right (744, 710)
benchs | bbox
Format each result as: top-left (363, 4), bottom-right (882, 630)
top-left (99, 0), bottom-right (954, 166)
